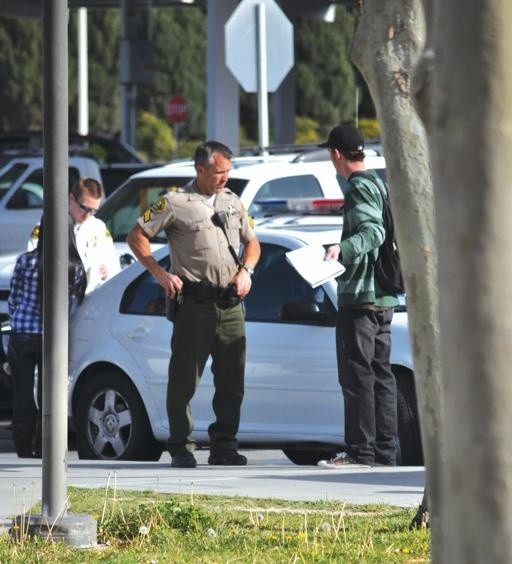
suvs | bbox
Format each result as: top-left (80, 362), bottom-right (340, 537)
top-left (0, 143), bottom-right (387, 370)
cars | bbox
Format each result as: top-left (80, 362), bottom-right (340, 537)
top-left (36, 197), bottom-right (422, 465)
top-left (0, 154), bottom-right (179, 259)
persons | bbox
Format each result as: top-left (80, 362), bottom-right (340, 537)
top-left (6, 208), bottom-right (90, 459)
top-left (124, 139), bottom-right (265, 468)
top-left (28, 176), bottom-right (121, 292)
top-left (316, 122), bottom-right (406, 470)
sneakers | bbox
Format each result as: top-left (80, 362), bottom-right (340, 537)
top-left (173, 447), bottom-right (197, 467)
top-left (316, 452), bottom-right (372, 470)
top-left (208, 451), bottom-right (247, 465)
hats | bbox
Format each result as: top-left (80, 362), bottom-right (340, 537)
top-left (318, 124), bottom-right (365, 151)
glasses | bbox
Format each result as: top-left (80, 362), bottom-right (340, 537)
top-left (78, 200), bottom-right (100, 215)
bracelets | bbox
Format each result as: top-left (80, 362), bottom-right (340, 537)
top-left (241, 263), bottom-right (254, 274)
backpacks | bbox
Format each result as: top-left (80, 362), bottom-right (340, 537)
top-left (347, 172), bottom-right (405, 297)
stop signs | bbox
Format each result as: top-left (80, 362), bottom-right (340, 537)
top-left (165, 95), bottom-right (188, 123)
